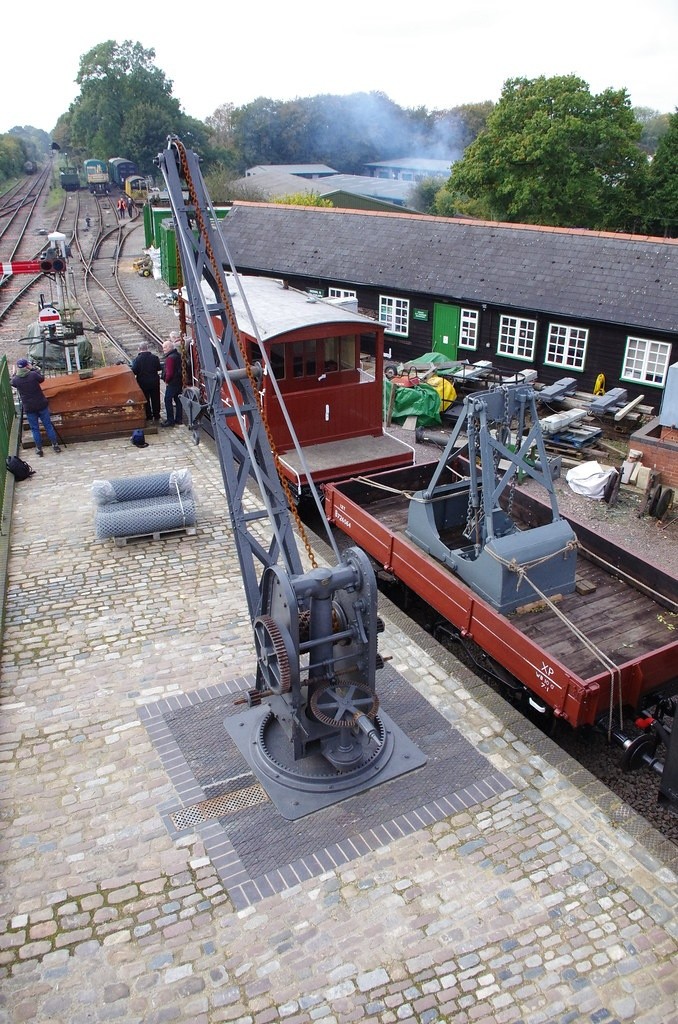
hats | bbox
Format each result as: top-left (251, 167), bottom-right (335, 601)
top-left (17, 359), bottom-right (28, 368)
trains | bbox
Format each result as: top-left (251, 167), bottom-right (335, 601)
top-left (109, 158), bottom-right (148, 204)
top-left (172, 276), bottom-right (678, 810)
top-left (84, 159), bottom-right (112, 195)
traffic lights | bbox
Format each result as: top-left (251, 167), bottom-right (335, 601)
top-left (40, 257), bottom-right (66, 272)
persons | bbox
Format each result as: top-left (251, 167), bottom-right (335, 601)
top-left (130, 341), bottom-right (162, 424)
top-left (117, 198), bottom-right (134, 218)
top-left (10, 359), bottom-right (61, 457)
top-left (159, 331), bottom-right (183, 426)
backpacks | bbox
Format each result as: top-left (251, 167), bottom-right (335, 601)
top-left (6, 455), bottom-right (36, 482)
top-left (129, 429), bottom-right (149, 448)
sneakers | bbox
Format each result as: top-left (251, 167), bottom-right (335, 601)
top-left (35, 447), bottom-right (43, 457)
top-left (160, 419), bottom-right (182, 428)
top-left (52, 444), bottom-right (61, 453)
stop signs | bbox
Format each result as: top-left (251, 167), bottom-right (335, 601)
top-left (38, 308), bottom-right (61, 324)
